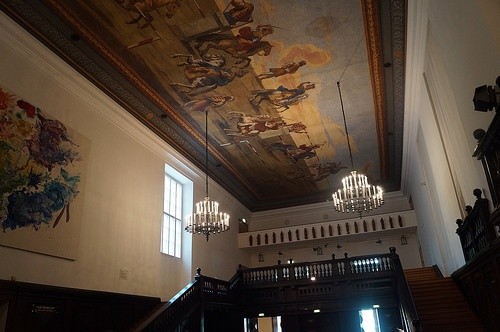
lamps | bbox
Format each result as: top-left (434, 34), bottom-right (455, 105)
top-left (331, 81), bottom-right (384, 219)
top-left (185, 111), bottom-right (231, 242)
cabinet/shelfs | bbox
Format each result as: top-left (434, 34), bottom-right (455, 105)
top-left (456, 188), bottom-right (496, 263)
